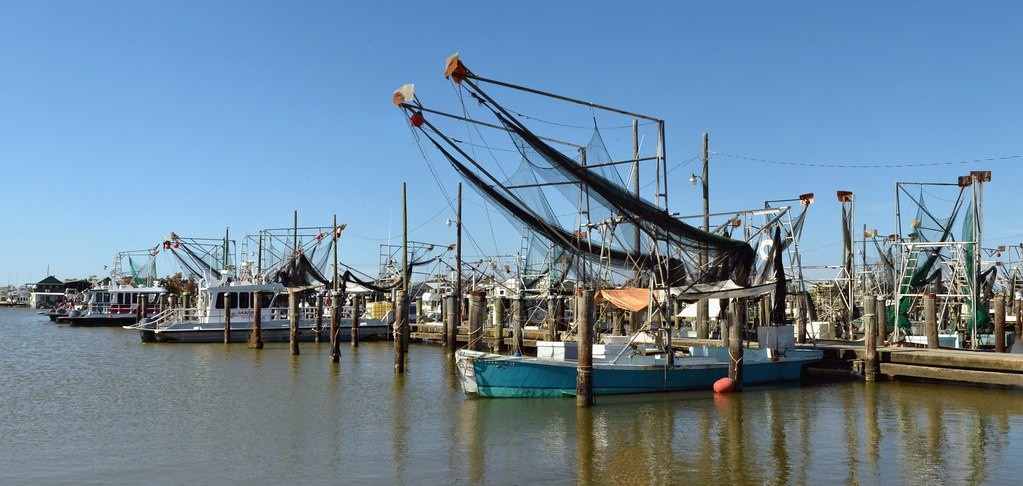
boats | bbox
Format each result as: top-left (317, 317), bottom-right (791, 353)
top-left (38, 169), bottom-right (1023, 352)
top-left (392, 52), bottom-right (823, 398)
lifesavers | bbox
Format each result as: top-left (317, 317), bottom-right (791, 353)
top-left (59, 302), bottom-right (66, 307)
top-left (77, 296), bottom-right (82, 301)
